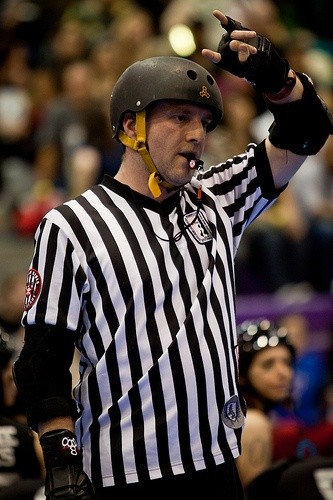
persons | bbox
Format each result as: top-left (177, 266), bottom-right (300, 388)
top-left (12, 10), bottom-right (333, 500)
top-left (0, 0), bottom-right (332, 500)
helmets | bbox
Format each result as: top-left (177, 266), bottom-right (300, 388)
top-left (109, 56), bottom-right (221, 137)
top-left (237, 317), bottom-right (292, 369)
